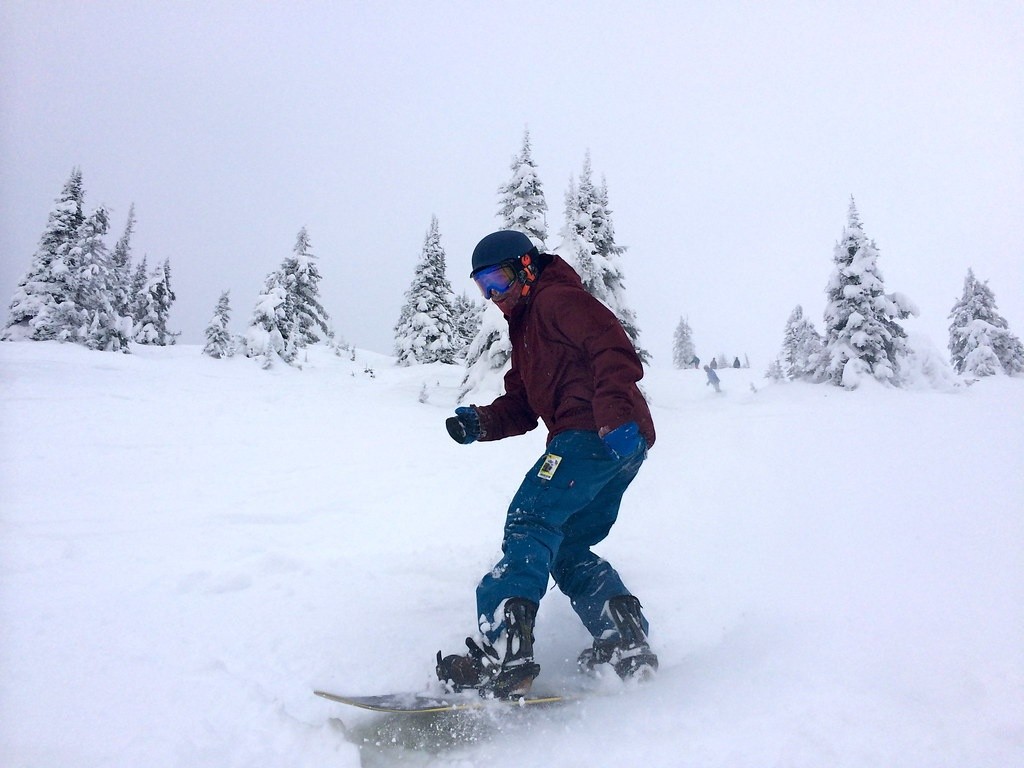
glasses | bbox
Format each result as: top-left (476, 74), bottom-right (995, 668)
top-left (471, 247), bottom-right (539, 299)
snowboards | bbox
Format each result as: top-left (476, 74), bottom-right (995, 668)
top-left (312, 690), bottom-right (579, 714)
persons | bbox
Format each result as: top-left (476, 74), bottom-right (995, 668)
top-left (439, 230), bottom-right (658, 702)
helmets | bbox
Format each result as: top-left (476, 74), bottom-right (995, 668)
top-left (472, 230), bottom-right (534, 270)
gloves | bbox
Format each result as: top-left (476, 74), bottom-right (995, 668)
top-left (601, 419), bottom-right (646, 461)
top-left (446, 406), bottom-right (481, 443)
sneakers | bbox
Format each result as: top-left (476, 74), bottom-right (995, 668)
top-left (443, 649), bottom-right (532, 695)
top-left (577, 650), bottom-right (655, 682)
